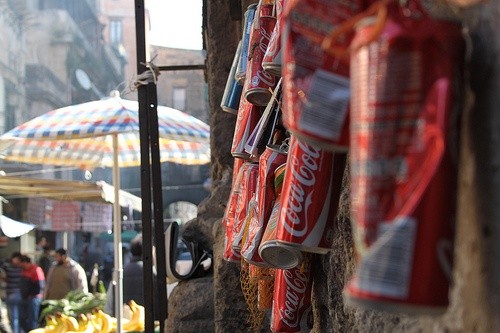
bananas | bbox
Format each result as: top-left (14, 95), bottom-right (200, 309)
top-left (27, 300), bottom-right (146, 333)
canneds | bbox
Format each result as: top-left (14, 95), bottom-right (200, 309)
top-left (341, 14), bottom-right (465, 314)
top-left (222, 0), bottom-right (372, 333)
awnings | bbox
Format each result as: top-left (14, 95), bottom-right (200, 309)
top-left (0, 174), bottom-right (143, 212)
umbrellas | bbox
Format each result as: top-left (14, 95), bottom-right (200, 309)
top-left (0, 89), bottom-right (211, 331)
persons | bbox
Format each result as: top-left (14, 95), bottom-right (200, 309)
top-left (0, 235), bottom-right (21, 268)
top-left (0, 302), bottom-right (14, 333)
top-left (43, 249), bottom-right (58, 262)
top-left (18, 256), bottom-right (46, 332)
top-left (32, 236), bottom-right (49, 265)
top-left (41, 248), bottom-right (90, 302)
top-left (88, 262), bottom-right (103, 293)
top-left (0, 252), bottom-right (23, 333)
top-left (105, 229), bottom-right (169, 332)
